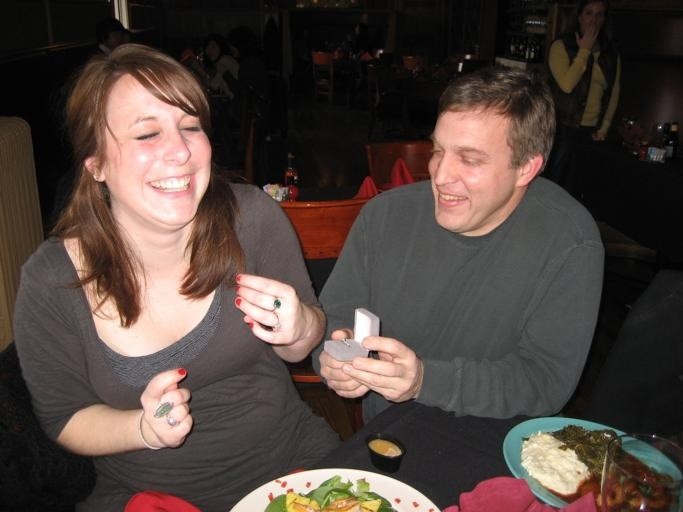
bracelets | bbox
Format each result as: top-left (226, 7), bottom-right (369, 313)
top-left (137, 410), bottom-right (160, 452)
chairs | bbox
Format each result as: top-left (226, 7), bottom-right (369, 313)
top-left (277, 198), bottom-right (372, 432)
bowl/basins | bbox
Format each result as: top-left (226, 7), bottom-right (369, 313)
top-left (311, 51), bottom-right (329, 65)
top-left (367, 432), bottom-right (406, 473)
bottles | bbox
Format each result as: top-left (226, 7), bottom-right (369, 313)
top-left (284, 153), bottom-right (299, 186)
top-left (638, 119), bottom-right (682, 164)
top-left (510, 35), bottom-right (540, 60)
top-left (471, 43), bottom-right (480, 60)
top-left (285, 176), bottom-right (300, 202)
top-left (334, 47), bottom-right (360, 63)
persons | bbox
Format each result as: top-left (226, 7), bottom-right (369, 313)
top-left (546, 1), bottom-right (625, 219)
top-left (301, 67), bottom-right (606, 427)
top-left (13, 43), bottom-right (342, 512)
top-left (164, 14), bottom-right (473, 188)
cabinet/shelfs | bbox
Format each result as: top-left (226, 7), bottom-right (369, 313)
top-left (495, 2), bottom-right (577, 70)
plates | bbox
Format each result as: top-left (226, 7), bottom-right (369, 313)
top-left (503, 417), bottom-right (682, 512)
top-left (228, 466), bottom-right (442, 512)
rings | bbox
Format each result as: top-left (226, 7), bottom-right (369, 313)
top-left (165, 415), bottom-right (175, 425)
top-left (271, 296), bottom-right (282, 313)
top-left (153, 401), bottom-right (172, 417)
top-left (341, 337), bottom-right (352, 351)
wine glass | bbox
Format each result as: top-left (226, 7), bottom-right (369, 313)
top-left (600, 432), bottom-right (683, 512)
